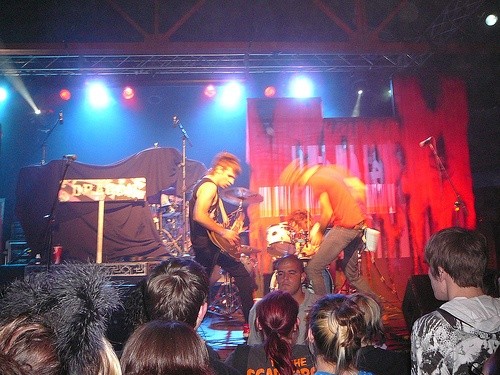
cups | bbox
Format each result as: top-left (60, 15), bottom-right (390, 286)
top-left (51, 244), bottom-right (64, 265)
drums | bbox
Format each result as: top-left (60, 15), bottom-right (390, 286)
top-left (361, 226), bottom-right (381, 255)
top-left (270, 257), bottom-right (334, 297)
top-left (264, 221), bottom-right (305, 257)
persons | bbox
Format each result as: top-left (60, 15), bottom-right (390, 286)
top-left (189, 151), bottom-right (255, 337)
top-left (269, 158), bottom-right (382, 313)
top-left (0, 228), bottom-right (500, 375)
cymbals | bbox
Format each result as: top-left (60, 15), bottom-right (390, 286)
top-left (219, 186), bottom-right (265, 207)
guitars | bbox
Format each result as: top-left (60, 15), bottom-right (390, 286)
top-left (207, 210), bottom-right (249, 263)
